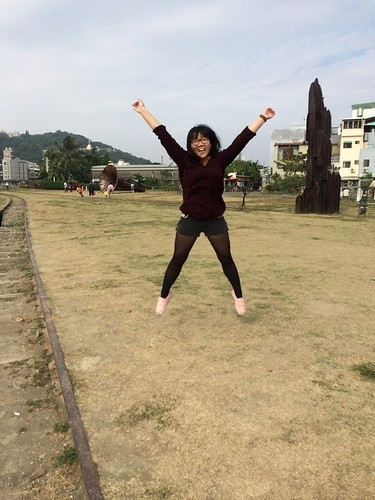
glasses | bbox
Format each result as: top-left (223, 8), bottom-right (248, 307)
top-left (190, 137), bottom-right (211, 145)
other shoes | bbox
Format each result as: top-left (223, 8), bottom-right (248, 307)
top-left (231, 289), bottom-right (245, 315)
top-left (156, 291), bottom-right (172, 314)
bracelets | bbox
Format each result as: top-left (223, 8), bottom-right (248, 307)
top-left (259, 114), bottom-right (267, 123)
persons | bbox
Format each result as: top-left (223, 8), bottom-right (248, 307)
top-left (3, 175), bottom-right (374, 213)
top-left (131, 98), bottom-right (278, 315)
